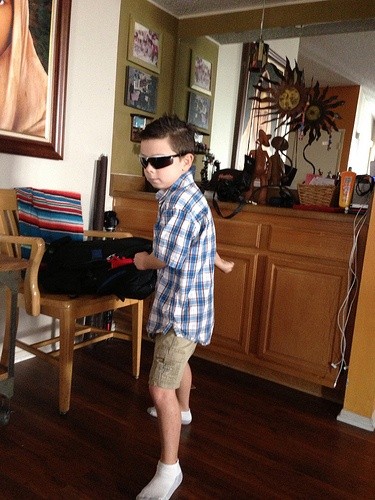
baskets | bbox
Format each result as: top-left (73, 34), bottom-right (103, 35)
top-left (297, 183), bottom-right (338, 208)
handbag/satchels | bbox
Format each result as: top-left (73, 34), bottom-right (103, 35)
top-left (29, 236), bottom-right (158, 302)
top-left (216, 168), bottom-right (244, 203)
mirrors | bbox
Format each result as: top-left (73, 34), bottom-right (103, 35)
top-left (170, 13), bottom-right (375, 196)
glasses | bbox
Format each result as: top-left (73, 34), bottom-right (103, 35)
top-left (140, 152), bottom-right (187, 170)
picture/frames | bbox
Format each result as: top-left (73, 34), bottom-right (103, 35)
top-left (190, 48), bottom-right (212, 97)
top-left (127, 14), bottom-right (162, 74)
top-left (0, 0), bottom-right (72, 161)
top-left (186, 92), bottom-right (212, 129)
top-left (125, 65), bottom-right (159, 114)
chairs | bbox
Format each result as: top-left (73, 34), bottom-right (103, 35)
top-left (0, 189), bottom-right (145, 418)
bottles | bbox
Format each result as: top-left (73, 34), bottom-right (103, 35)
top-left (338, 166), bottom-right (356, 208)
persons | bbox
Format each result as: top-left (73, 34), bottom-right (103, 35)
top-left (134, 27), bottom-right (158, 64)
top-left (133, 110), bottom-right (234, 500)
top-left (195, 58), bottom-right (211, 90)
top-left (191, 94), bottom-right (208, 126)
top-left (0, 0), bottom-right (47, 137)
top-left (129, 69), bottom-right (155, 110)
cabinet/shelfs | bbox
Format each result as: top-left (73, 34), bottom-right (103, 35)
top-left (106, 193), bottom-right (364, 399)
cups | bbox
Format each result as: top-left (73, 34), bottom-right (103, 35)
top-left (104, 211), bottom-right (120, 233)
